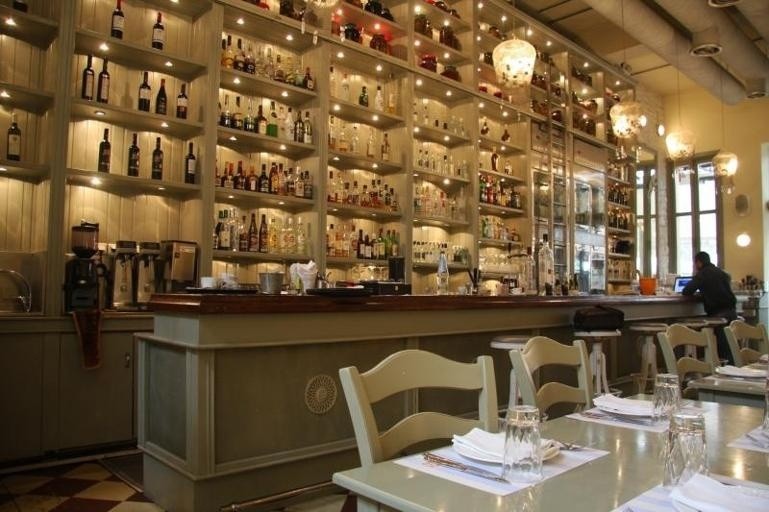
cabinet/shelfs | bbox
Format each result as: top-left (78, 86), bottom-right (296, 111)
top-left (0, 1), bottom-right (641, 474)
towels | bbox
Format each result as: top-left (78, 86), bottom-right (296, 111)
top-left (747, 423), bottom-right (769, 449)
top-left (666, 471), bottom-right (769, 512)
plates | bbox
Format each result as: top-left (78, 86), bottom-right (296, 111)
top-left (596, 398), bottom-right (653, 421)
top-left (452, 432), bottom-right (561, 465)
top-left (715, 366), bottom-right (766, 378)
top-left (672, 481), bottom-right (767, 512)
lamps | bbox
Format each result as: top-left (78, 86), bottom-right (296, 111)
top-left (662, 20), bottom-right (697, 179)
top-left (711, 8), bottom-right (738, 196)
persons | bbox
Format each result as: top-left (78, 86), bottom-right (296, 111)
top-left (682, 251), bottom-right (738, 367)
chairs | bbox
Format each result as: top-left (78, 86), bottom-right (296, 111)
top-left (724, 320), bottom-right (769, 367)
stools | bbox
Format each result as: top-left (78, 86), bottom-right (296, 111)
top-left (490, 314), bottom-right (746, 421)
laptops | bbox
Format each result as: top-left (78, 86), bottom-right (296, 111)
top-left (673, 277), bottom-right (701, 294)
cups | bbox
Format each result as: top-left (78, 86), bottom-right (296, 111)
top-left (660, 409), bottom-right (711, 488)
top-left (218, 273), bottom-right (238, 290)
top-left (200, 276), bottom-right (217, 289)
top-left (763, 376), bottom-right (769, 427)
top-left (457, 286), bottom-right (467, 296)
top-left (502, 404), bottom-right (545, 486)
top-left (651, 374), bottom-right (682, 422)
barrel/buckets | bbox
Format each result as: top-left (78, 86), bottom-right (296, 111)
top-left (258, 271), bottom-right (286, 294)
top-left (639, 277), bottom-right (657, 295)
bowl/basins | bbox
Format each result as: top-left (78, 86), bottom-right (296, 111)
top-left (98, 128), bottom-right (197, 184)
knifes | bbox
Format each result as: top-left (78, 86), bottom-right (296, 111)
top-left (422, 449), bottom-right (513, 487)
top-left (744, 434), bottom-right (765, 448)
top-left (582, 410), bottom-right (647, 425)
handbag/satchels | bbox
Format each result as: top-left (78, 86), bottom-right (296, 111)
top-left (573, 303), bottom-right (624, 332)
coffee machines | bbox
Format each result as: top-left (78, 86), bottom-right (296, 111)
top-left (64, 218), bottom-right (102, 313)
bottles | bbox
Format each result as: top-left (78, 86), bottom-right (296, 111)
top-left (81, 51), bottom-right (189, 120)
top-left (410, 103), bottom-right (474, 223)
top-left (326, 65), bottom-right (403, 260)
top-left (536, 233), bottom-right (556, 295)
top-left (742, 275), bottom-right (757, 291)
top-left (110, 0), bottom-right (126, 39)
top-left (479, 116), bottom-right (524, 241)
top-left (152, 12), bottom-right (166, 51)
top-left (608, 181), bottom-right (634, 279)
top-left (315, 272), bottom-right (323, 289)
top-left (408, 238), bottom-right (470, 263)
top-left (6, 110), bottom-right (22, 162)
top-left (209, 35), bottom-right (315, 257)
top-left (478, 241), bottom-right (578, 298)
top-left (435, 251), bottom-right (449, 295)
